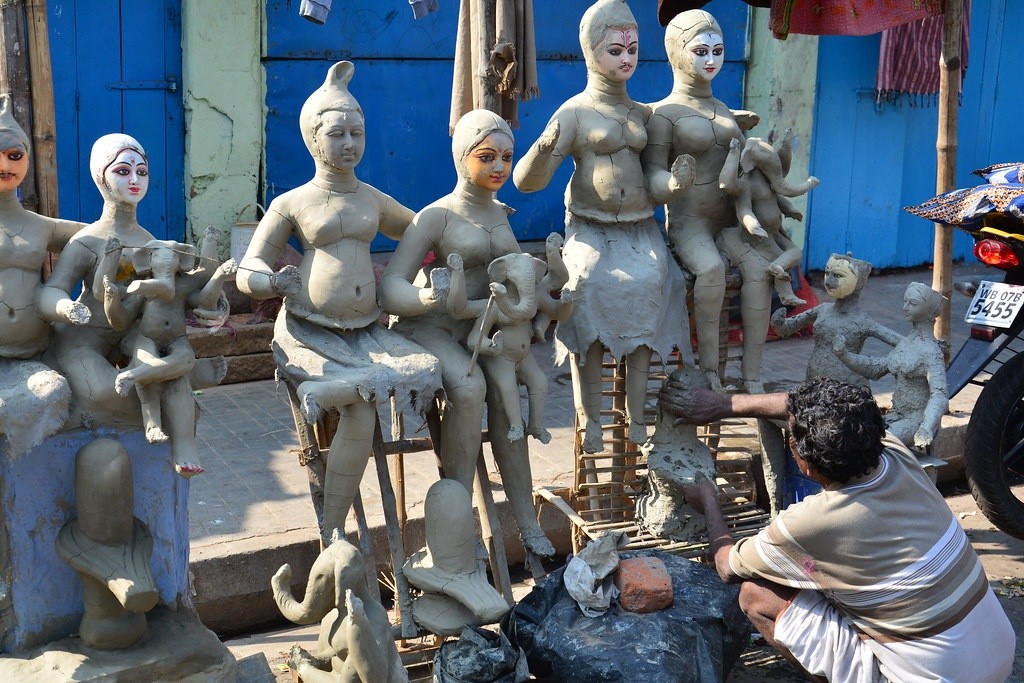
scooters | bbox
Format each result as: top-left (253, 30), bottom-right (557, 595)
top-left (897, 160), bottom-right (1024, 545)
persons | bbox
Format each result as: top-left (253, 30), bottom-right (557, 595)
top-left (0, 91), bottom-right (238, 477)
top-left (521, 0), bottom-right (821, 454)
top-left (234, 59), bottom-right (575, 558)
top-left (656, 377), bottom-right (1016, 683)
top-left (771, 250), bottom-right (949, 448)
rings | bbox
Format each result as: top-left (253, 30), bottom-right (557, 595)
top-left (682, 496), bottom-right (687, 504)
